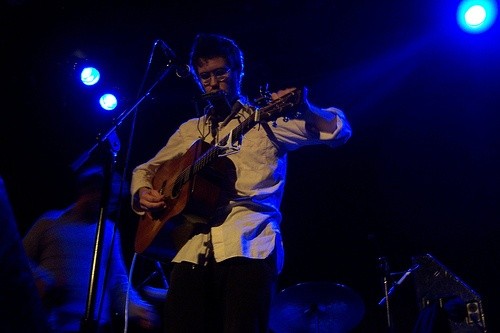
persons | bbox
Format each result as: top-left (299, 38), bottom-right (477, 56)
top-left (130, 34), bottom-right (353, 333)
top-left (21, 164), bottom-right (154, 333)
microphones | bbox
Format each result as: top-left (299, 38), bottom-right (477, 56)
top-left (157, 40), bottom-right (190, 77)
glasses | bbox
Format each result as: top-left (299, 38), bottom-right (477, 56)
top-left (200, 68), bottom-right (233, 85)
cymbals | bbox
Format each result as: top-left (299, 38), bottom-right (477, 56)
top-left (267, 282), bottom-right (366, 333)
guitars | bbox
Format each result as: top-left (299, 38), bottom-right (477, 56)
top-left (134, 85), bottom-right (308, 259)
